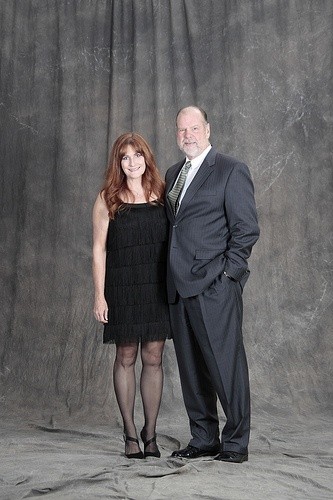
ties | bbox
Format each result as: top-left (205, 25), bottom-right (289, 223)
top-left (166, 160), bottom-right (192, 218)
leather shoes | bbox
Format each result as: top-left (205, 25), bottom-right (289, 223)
top-left (214, 449), bottom-right (251, 464)
top-left (169, 443), bottom-right (221, 460)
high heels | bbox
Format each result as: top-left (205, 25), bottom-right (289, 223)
top-left (122, 433), bottom-right (144, 461)
top-left (141, 425), bottom-right (163, 458)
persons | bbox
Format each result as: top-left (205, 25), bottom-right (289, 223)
top-left (162, 106), bottom-right (261, 464)
top-left (88, 131), bottom-right (184, 454)
